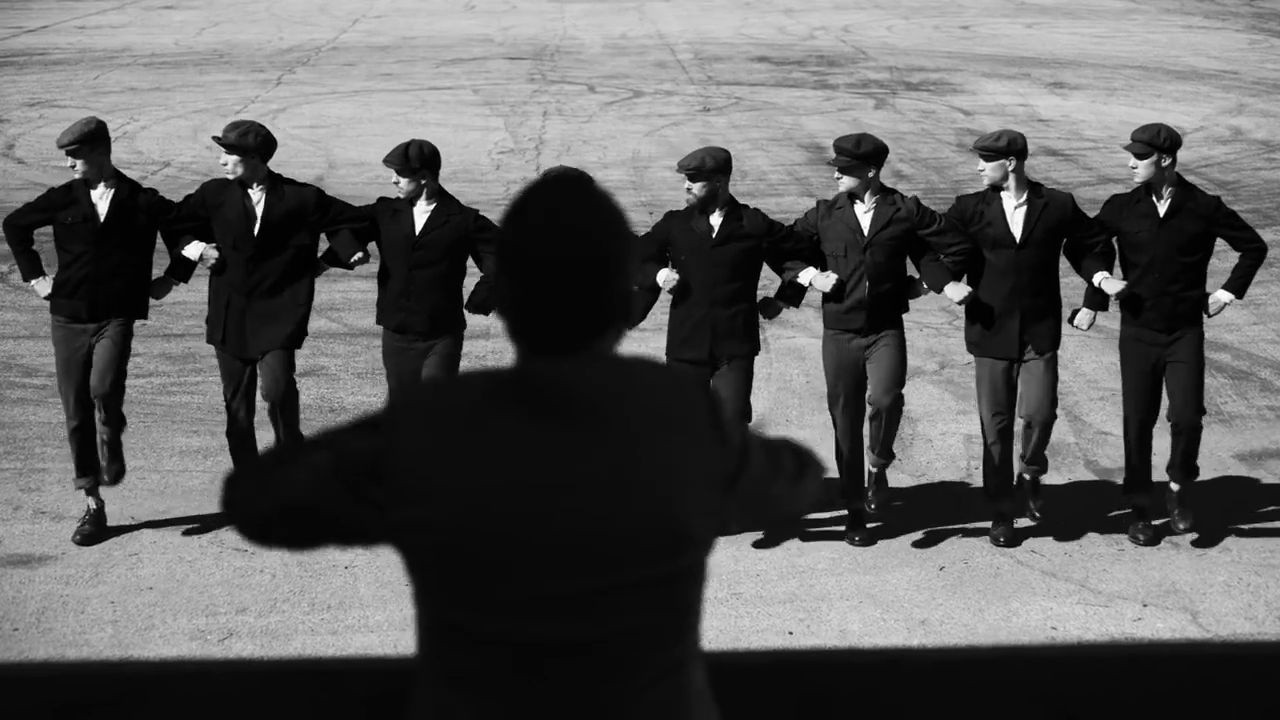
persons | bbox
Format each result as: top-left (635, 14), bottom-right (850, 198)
top-left (906, 131), bottom-right (1116, 547)
top-left (1061, 122), bottom-right (1267, 545)
top-left (630, 146), bottom-right (812, 507)
top-left (762, 132), bottom-right (973, 547)
top-left (2, 116), bottom-right (207, 547)
top-left (162, 120), bottom-right (371, 471)
top-left (316, 140), bottom-right (508, 406)
top-left (221, 165), bottom-right (822, 720)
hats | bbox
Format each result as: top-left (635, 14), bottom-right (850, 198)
top-left (381, 139), bottom-right (441, 176)
top-left (56, 116), bottom-right (109, 150)
top-left (825, 133), bottom-right (890, 166)
top-left (968, 130), bottom-right (1027, 161)
top-left (676, 147), bottom-right (732, 172)
top-left (1122, 122), bottom-right (1182, 156)
top-left (213, 120), bottom-right (279, 162)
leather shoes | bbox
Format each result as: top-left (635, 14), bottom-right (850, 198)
top-left (101, 433), bottom-right (126, 485)
top-left (1168, 483), bottom-right (1195, 533)
top-left (1016, 474), bottom-right (1049, 522)
top-left (988, 506), bottom-right (1014, 547)
top-left (1127, 506), bottom-right (1154, 547)
top-left (72, 500), bottom-right (107, 546)
top-left (846, 511), bottom-right (868, 545)
top-left (865, 467), bottom-right (888, 513)
